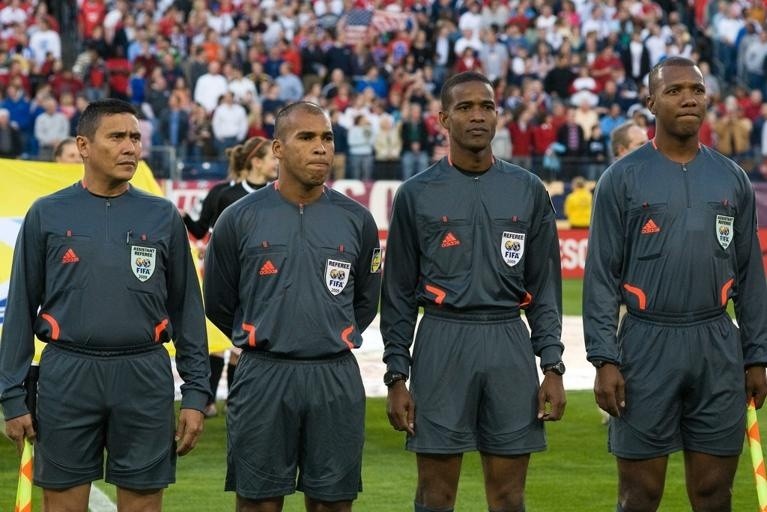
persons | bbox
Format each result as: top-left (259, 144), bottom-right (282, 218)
top-left (1, 100), bottom-right (215, 512)
top-left (173, 137), bottom-right (277, 418)
top-left (204, 100), bottom-right (383, 512)
top-left (583, 57), bottom-right (767, 510)
top-left (381, 71), bottom-right (567, 512)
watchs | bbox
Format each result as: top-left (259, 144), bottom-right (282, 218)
top-left (592, 357), bottom-right (606, 368)
top-left (383, 370), bottom-right (407, 387)
top-left (540, 361), bottom-right (566, 376)
